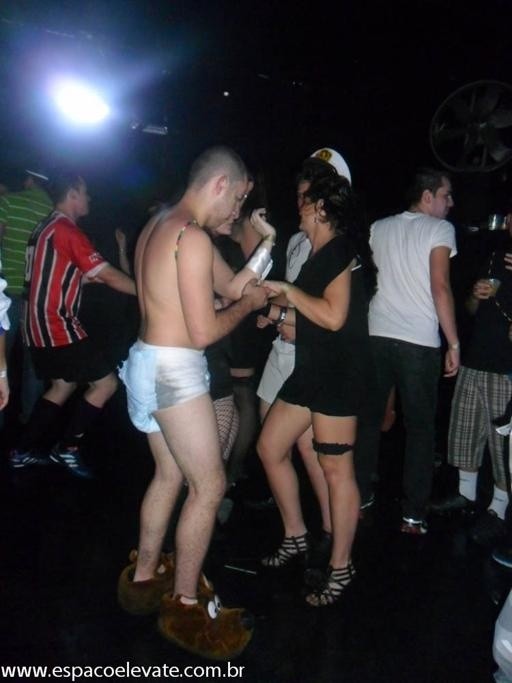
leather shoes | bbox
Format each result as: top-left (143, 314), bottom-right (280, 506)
top-left (435, 494), bottom-right (480, 515)
top-left (468, 512), bottom-right (508, 546)
top-left (479, 554), bottom-right (512, 609)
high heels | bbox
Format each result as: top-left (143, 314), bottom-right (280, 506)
top-left (263, 533), bottom-right (316, 569)
top-left (305, 566), bottom-right (359, 607)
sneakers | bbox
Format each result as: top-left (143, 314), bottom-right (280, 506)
top-left (158, 590), bottom-right (258, 662)
top-left (116, 551), bottom-right (219, 617)
top-left (11, 448), bottom-right (55, 470)
top-left (403, 517), bottom-right (431, 534)
top-left (49, 446), bottom-right (96, 481)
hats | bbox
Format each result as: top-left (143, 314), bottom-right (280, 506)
top-left (309, 146), bottom-right (354, 187)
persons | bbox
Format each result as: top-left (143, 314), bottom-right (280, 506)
top-left (1, 146), bottom-right (510, 683)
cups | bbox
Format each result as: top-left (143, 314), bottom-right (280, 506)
top-left (488, 213), bottom-right (500, 231)
top-left (483, 278), bottom-right (501, 298)
